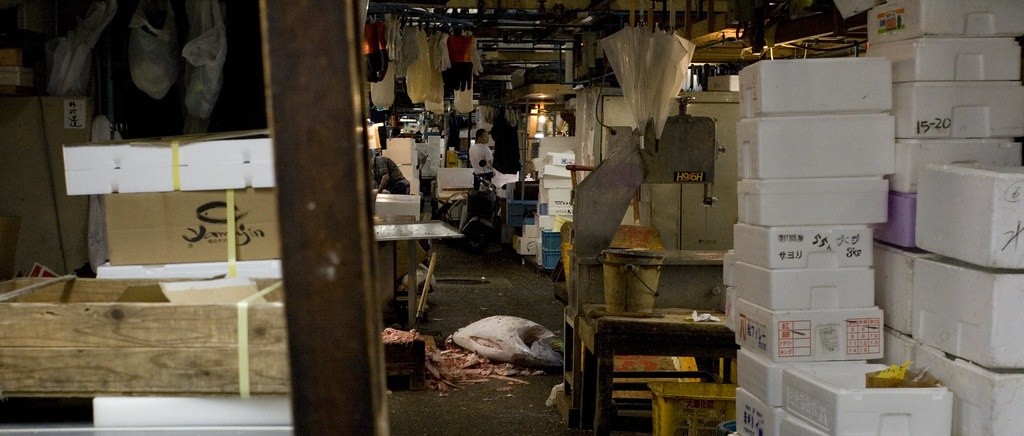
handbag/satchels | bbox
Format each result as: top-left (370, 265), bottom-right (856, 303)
top-left (182, 0), bottom-right (227, 120)
top-left (128, 0), bottom-right (179, 100)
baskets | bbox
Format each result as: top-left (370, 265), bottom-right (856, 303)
top-left (467, 188), bottom-right (496, 219)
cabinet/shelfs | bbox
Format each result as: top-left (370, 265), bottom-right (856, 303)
top-left (574, 87), bottom-right (741, 311)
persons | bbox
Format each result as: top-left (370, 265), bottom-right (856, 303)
top-left (369, 149), bottom-right (410, 200)
top-left (469, 128), bottom-right (494, 191)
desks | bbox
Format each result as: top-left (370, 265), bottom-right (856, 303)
top-left (563, 309), bottom-right (736, 436)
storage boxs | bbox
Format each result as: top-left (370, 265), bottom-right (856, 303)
top-left (707, 0), bottom-right (1024, 436)
top-left (376, 134), bottom-right (575, 267)
top-left (0, 49), bottom-right (298, 436)
top-left (646, 380), bottom-right (737, 436)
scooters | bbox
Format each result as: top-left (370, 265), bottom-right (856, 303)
top-left (432, 159), bottom-right (507, 253)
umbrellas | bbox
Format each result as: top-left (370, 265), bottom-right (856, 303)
top-left (600, 0), bottom-right (696, 153)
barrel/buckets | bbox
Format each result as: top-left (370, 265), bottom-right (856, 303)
top-left (602, 248), bottom-right (664, 315)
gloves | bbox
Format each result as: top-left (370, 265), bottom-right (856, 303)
top-left (365, 14), bottom-right (483, 114)
